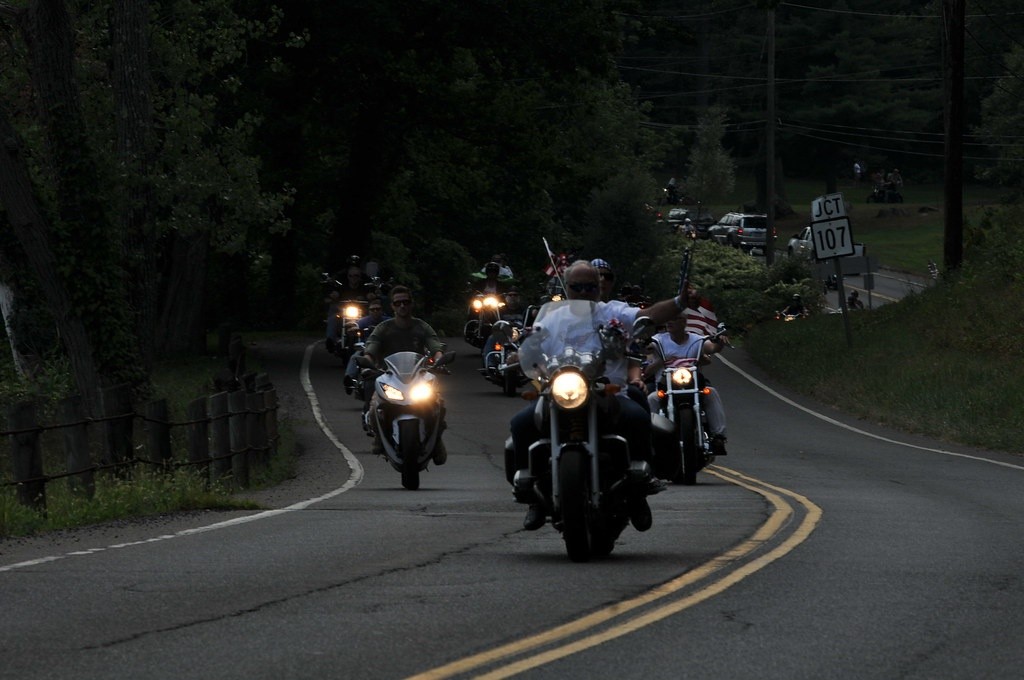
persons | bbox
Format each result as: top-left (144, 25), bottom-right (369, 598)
top-left (679, 218), bottom-right (700, 236)
top-left (504, 259), bottom-right (701, 532)
top-left (848, 290), bottom-right (860, 308)
top-left (474, 254), bottom-right (537, 372)
top-left (644, 311), bottom-right (730, 455)
top-left (362, 286), bottom-right (448, 465)
top-left (782, 294), bottom-right (807, 315)
top-left (326, 255), bottom-right (373, 353)
top-left (344, 300), bottom-right (392, 395)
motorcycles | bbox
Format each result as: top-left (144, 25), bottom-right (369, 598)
top-left (639, 321), bottom-right (735, 486)
top-left (462, 279), bottom-right (646, 399)
top-left (351, 349), bottom-right (457, 492)
top-left (319, 269), bottom-right (392, 402)
top-left (502, 299), bottom-right (675, 565)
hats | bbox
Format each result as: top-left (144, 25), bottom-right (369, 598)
top-left (591, 258), bottom-right (610, 269)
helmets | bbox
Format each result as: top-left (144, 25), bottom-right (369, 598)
top-left (486, 261), bottom-right (501, 278)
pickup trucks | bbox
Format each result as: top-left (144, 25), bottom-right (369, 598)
top-left (789, 226), bottom-right (864, 278)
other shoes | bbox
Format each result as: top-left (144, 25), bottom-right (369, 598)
top-left (429, 438), bottom-right (446, 466)
top-left (708, 434), bottom-right (729, 457)
top-left (626, 493), bottom-right (654, 532)
top-left (370, 437), bottom-right (385, 457)
top-left (524, 502), bottom-right (551, 531)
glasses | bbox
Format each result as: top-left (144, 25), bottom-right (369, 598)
top-left (508, 293), bottom-right (519, 297)
top-left (598, 272), bottom-right (614, 281)
top-left (669, 316), bottom-right (681, 322)
top-left (393, 299), bottom-right (412, 307)
top-left (370, 308), bottom-right (381, 312)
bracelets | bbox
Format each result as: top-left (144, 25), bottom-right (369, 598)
top-left (675, 297), bottom-right (686, 310)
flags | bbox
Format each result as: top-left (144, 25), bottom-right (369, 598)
top-left (544, 254), bottom-right (566, 278)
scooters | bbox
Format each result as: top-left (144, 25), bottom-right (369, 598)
top-left (684, 229), bottom-right (697, 241)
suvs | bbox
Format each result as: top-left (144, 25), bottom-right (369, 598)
top-left (659, 207), bottom-right (716, 240)
top-left (707, 212), bottom-right (778, 254)
